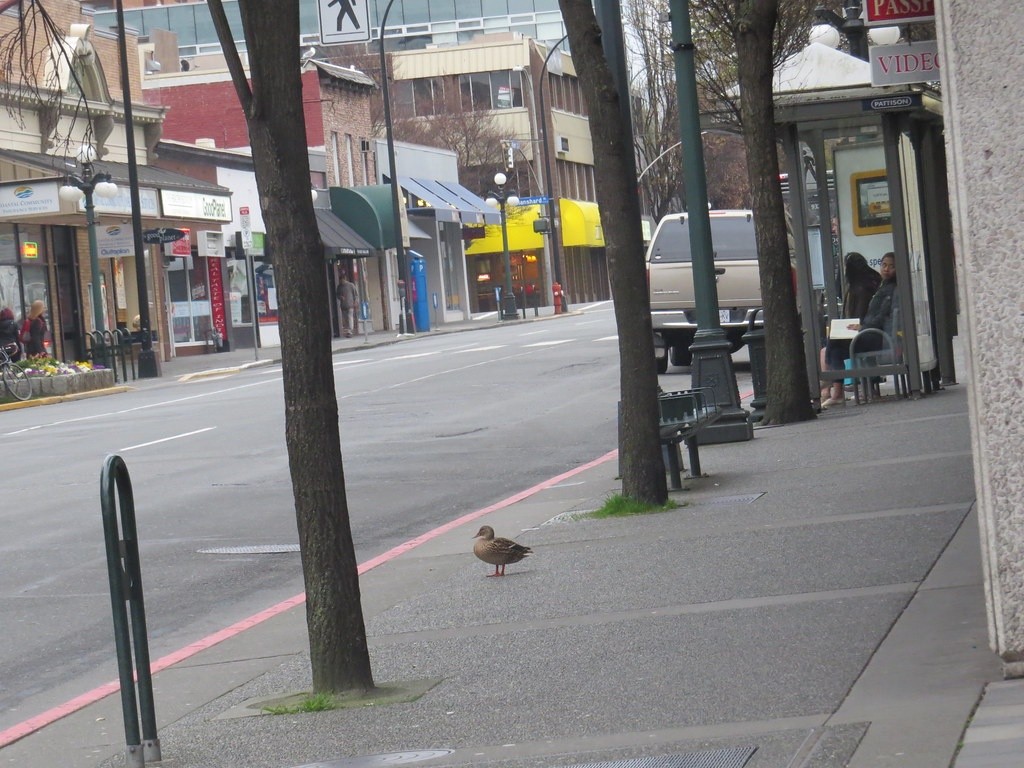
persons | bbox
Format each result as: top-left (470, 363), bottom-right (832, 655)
top-left (337, 274), bottom-right (360, 338)
top-left (0, 309), bottom-right (22, 368)
top-left (24, 301), bottom-right (49, 360)
top-left (821, 252), bottom-right (897, 408)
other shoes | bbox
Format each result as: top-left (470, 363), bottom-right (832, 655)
top-left (820, 395), bottom-right (845, 408)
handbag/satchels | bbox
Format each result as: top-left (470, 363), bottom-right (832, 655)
top-left (0, 341), bottom-right (20, 359)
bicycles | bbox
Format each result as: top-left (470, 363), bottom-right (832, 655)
top-left (0, 345), bottom-right (32, 401)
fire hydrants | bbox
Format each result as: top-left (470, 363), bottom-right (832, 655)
top-left (552, 282), bottom-right (564, 314)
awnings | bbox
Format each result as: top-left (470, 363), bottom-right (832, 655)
top-left (330, 183), bottom-right (397, 250)
top-left (314, 209), bottom-right (376, 261)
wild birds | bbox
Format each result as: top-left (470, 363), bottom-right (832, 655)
top-left (470, 525), bottom-right (536, 578)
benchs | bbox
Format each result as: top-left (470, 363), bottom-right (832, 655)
top-left (849, 308), bottom-right (909, 405)
top-left (656, 382), bottom-right (723, 491)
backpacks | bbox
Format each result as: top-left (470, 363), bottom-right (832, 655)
top-left (20, 318), bottom-right (44, 344)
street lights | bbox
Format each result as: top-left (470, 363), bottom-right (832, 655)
top-left (512, 66), bottom-right (554, 306)
top-left (484, 172), bottom-right (519, 320)
top-left (59, 147), bottom-right (117, 382)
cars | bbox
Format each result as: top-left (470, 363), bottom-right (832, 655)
top-left (648, 209), bottom-right (804, 375)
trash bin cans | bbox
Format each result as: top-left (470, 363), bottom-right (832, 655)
top-left (92, 339), bottom-right (118, 381)
top-left (741, 308), bottom-right (769, 422)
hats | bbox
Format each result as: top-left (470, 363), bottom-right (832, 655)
top-left (0, 308), bottom-right (14, 319)
